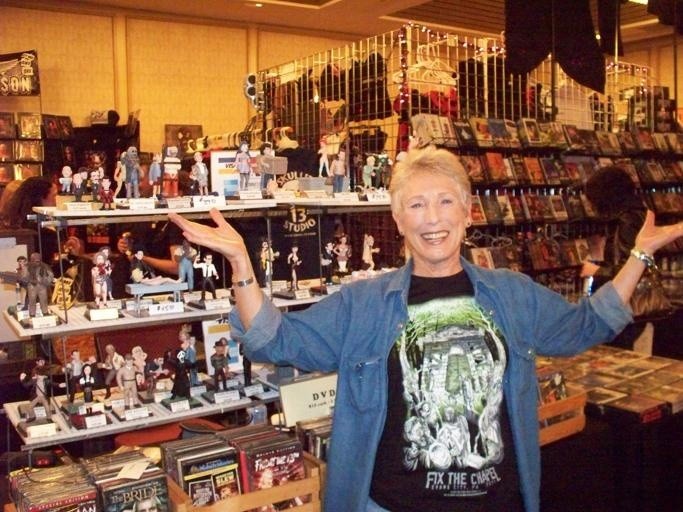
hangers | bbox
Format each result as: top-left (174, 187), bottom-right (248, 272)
top-left (392, 44), bottom-right (461, 88)
top-left (184, 127), bottom-right (300, 160)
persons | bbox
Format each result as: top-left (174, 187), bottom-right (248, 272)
top-left (256, 467), bottom-right (293, 512)
top-left (579, 166), bottom-right (659, 349)
top-left (134, 496), bottom-right (160, 512)
top-left (14, 109), bottom-right (415, 425)
top-left (168, 146), bottom-right (683, 511)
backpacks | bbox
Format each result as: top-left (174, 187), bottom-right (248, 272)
top-left (612, 207), bottom-right (675, 324)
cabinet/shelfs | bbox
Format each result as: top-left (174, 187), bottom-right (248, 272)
top-left (2, 189), bottom-right (407, 446)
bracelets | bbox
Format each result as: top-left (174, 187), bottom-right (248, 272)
top-left (630, 246), bottom-right (656, 269)
